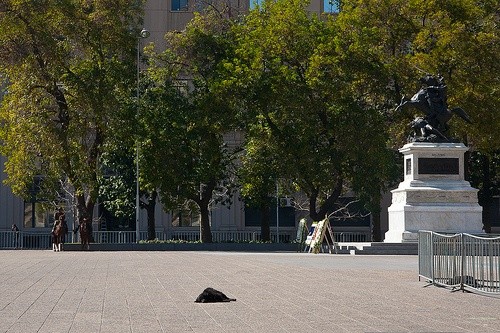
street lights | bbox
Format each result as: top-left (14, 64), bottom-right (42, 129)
top-left (135, 27), bottom-right (150, 246)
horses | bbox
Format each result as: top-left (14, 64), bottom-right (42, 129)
top-left (50, 212), bottom-right (66, 251)
top-left (73, 217), bottom-right (91, 251)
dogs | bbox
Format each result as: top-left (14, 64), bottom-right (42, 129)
top-left (195, 288), bottom-right (236, 303)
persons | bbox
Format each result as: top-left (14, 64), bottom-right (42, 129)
top-left (400, 77), bottom-right (437, 138)
top-left (12, 224), bottom-right (19, 247)
top-left (78, 212), bottom-right (91, 251)
top-left (52, 208), bottom-right (67, 251)
